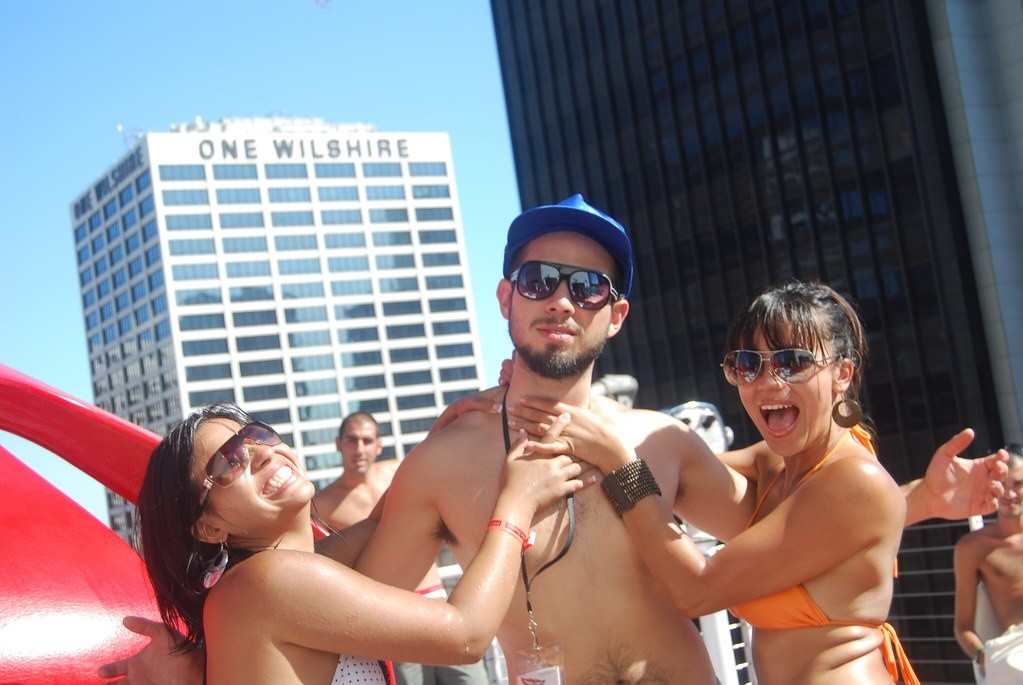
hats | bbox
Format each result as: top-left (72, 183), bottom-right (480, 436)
top-left (503, 193), bottom-right (634, 299)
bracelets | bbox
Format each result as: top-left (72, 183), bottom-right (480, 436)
top-left (600, 458), bottom-right (662, 519)
top-left (488, 519), bottom-right (536, 553)
top-left (976, 646), bottom-right (985, 664)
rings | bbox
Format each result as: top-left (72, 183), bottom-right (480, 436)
top-left (567, 464), bottom-right (574, 479)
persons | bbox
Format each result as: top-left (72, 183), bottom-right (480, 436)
top-left (99, 194), bottom-right (1023, 685)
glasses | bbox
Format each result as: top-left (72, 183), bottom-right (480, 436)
top-left (509, 260), bottom-right (619, 310)
top-left (987, 444), bottom-right (1023, 455)
top-left (720, 348), bottom-right (846, 386)
top-left (198, 420), bottom-right (283, 512)
